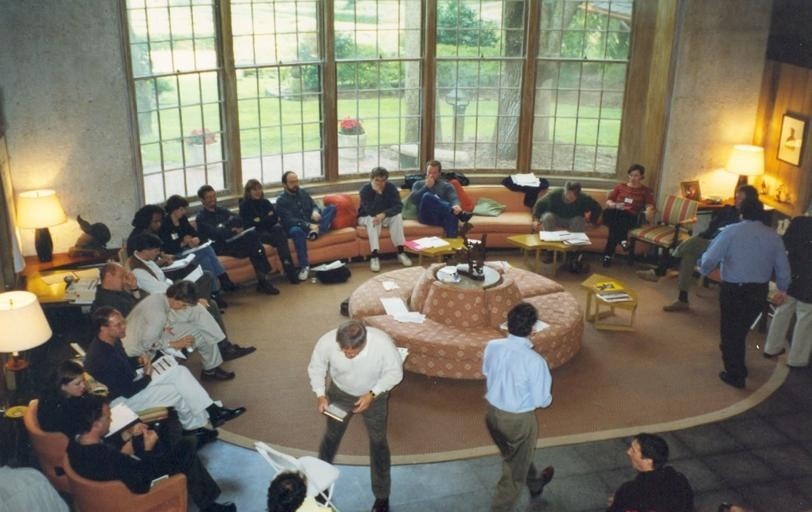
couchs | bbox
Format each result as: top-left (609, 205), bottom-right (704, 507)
top-left (358, 182), bottom-right (654, 288)
top-left (131, 190), bottom-right (359, 299)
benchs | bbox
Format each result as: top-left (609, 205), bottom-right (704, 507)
top-left (347, 258), bottom-right (586, 387)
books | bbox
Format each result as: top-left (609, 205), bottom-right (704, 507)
top-left (499, 318), bottom-right (550, 335)
top-left (324, 405), bottom-right (349, 422)
top-left (593, 281), bottom-right (623, 293)
top-left (539, 230), bottom-right (591, 246)
top-left (596, 292), bottom-right (633, 303)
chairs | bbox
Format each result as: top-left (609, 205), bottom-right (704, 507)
top-left (16, 399), bottom-right (71, 498)
top-left (52, 443), bottom-right (195, 511)
top-left (253, 437), bottom-right (343, 511)
top-left (128, 398), bottom-right (184, 450)
top-left (632, 196), bottom-right (706, 275)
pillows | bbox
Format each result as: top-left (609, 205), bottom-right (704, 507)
top-left (449, 179), bottom-right (474, 215)
top-left (399, 188), bottom-right (419, 222)
top-left (472, 196), bottom-right (507, 219)
top-left (321, 193), bottom-right (360, 229)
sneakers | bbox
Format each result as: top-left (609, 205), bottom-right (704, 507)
top-left (663, 299), bottom-right (689, 310)
top-left (307, 231), bottom-right (318, 240)
top-left (398, 253), bottom-right (412, 266)
top-left (371, 257), bottom-right (380, 272)
top-left (637, 269), bottom-right (659, 283)
top-left (460, 213), bottom-right (471, 222)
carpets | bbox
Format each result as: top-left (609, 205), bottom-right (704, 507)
top-left (183, 237), bottom-right (795, 472)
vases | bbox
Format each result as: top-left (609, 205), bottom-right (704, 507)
top-left (336, 135), bottom-right (367, 161)
top-left (187, 145), bottom-right (222, 169)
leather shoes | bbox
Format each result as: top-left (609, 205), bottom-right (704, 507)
top-left (222, 344), bottom-right (255, 361)
top-left (531, 466), bottom-right (553, 495)
top-left (603, 256), bottom-right (610, 265)
top-left (372, 497), bottom-right (390, 511)
top-left (183, 427), bottom-right (218, 439)
top-left (259, 255), bottom-right (309, 295)
top-left (210, 407), bottom-right (244, 427)
top-left (221, 282), bottom-right (239, 291)
top-left (623, 241), bottom-right (631, 251)
top-left (212, 293), bottom-right (227, 307)
top-left (719, 372), bottom-right (745, 388)
top-left (201, 369), bottom-right (234, 380)
top-left (765, 349), bottom-right (785, 357)
top-left (216, 503), bottom-right (237, 512)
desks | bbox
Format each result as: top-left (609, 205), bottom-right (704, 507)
top-left (695, 196), bottom-right (728, 220)
top-left (579, 273), bottom-right (640, 334)
top-left (505, 231), bottom-right (580, 278)
top-left (17, 245), bottom-right (123, 277)
top-left (403, 237), bottom-right (475, 267)
top-left (389, 141), bottom-right (469, 169)
top-left (20, 270), bottom-right (102, 327)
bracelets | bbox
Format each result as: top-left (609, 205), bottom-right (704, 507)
top-left (370, 391), bottom-right (376, 399)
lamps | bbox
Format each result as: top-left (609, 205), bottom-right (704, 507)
top-left (724, 144), bottom-right (768, 197)
top-left (0, 287), bottom-right (56, 400)
top-left (16, 188), bottom-right (69, 264)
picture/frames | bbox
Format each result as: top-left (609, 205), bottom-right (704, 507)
top-left (774, 112), bottom-right (807, 170)
top-left (681, 181), bottom-right (703, 201)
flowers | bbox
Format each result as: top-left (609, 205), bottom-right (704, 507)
top-left (183, 128), bottom-right (219, 144)
top-left (339, 116), bottom-right (365, 135)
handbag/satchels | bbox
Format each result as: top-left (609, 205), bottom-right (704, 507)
top-left (317, 267), bottom-right (350, 284)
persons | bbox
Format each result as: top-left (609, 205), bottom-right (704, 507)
top-left (531, 178), bottom-right (603, 273)
top-left (408, 159), bottom-right (472, 263)
top-left (634, 183), bottom-right (759, 313)
top-left (481, 303), bottom-right (555, 511)
top-left (760, 216), bottom-right (811, 368)
top-left (38, 170), bottom-right (337, 511)
top-left (308, 320), bottom-right (403, 510)
top-left (608, 432), bottom-right (696, 511)
top-left (0, 441), bottom-right (71, 511)
top-left (356, 167), bottom-right (412, 272)
top-left (599, 163), bottom-right (654, 267)
top-left (695, 196), bottom-right (792, 389)
